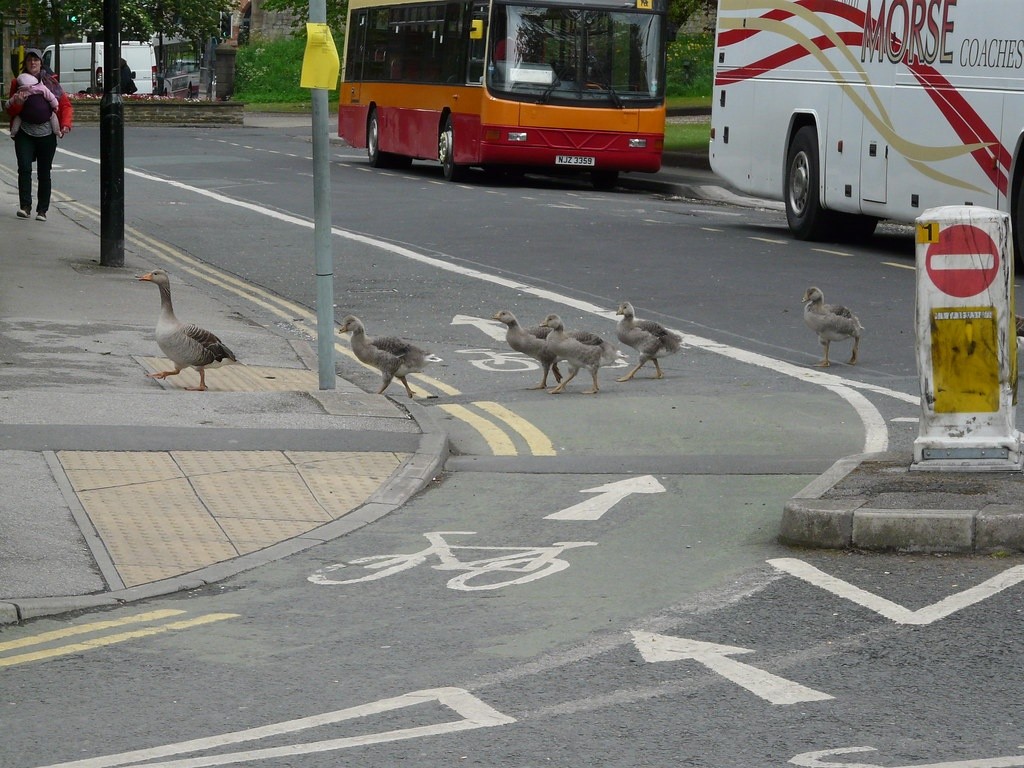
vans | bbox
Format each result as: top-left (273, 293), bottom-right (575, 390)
top-left (40, 41), bottom-right (158, 97)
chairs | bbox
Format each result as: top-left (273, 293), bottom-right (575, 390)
top-left (375, 49), bottom-right (440, 83)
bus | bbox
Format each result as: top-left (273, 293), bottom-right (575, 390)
top-left (708, 0), bottom-right (1024, 280)
top-left (337, 0), bottom-right (676, 191)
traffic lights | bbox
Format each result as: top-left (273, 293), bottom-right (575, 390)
top-left (219, 12), bottom-right (233, 39)
top-left (66, 14), bottom-right (82, 26)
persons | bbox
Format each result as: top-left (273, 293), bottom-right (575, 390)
top-left (494, 26), bottom-right (522, 62)
top-left (7, 48), bottom-right (75, 221)
top-left (8, 72), bottom-right (66, 139)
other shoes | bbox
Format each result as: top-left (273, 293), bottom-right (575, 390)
top-left (17, 209), bottom-right (30, 218)
top-left (36, 212), bottom-right (46, 220)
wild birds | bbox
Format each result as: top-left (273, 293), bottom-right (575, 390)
top-left (539, 313), bottom-right (627, 394)
top-left (800, 286), bottom-right (865, 367)
top-left (135, 270), bottom-right (247, 389)
top-left (614, 301), bottom-right (685, 382)
top-left (338, 315), bottom-right (437, 398)
top-left (492, 310), bottom-right (563, 391)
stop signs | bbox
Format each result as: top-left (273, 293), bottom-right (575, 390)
top-left (924, 224), bottom-right (1000, 298)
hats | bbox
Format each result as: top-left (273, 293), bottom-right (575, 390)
top-left (25, 48), bottom-right (43, 60)
